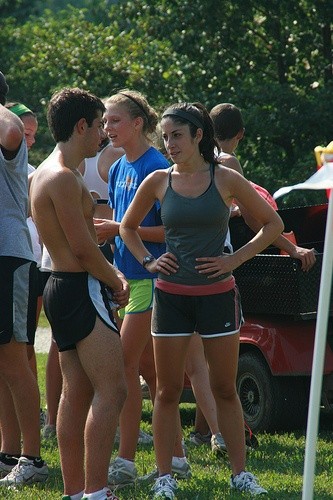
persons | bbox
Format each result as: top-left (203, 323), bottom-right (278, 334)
top-left (190, 103), bottom-right (316, 443)
top-left (30, 87), bottom-right (130, 500)
top-left (120, 103), bottom-right (285, 500)
top-left (93, 89), bottom-right (195, 484)
top-left (0, 69), bottom-right (51, 482)
top-left (5, 103), bottom-right (224, 455)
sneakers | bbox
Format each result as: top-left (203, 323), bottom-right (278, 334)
top-left (115, 426), bottom-right (153, 443)
top-left (230, 471), bottom-right (268, 500)
top-left (62, 488), bottom-right (84, 500)
top-left (151, 474), bottom-right (179, 500)
top-left (137, 461), bottom-right (192, 488)
top-left (210, 433), bottom-right (255, 460)
top-left (81, 487), bottom-right (120, 500)
top-left (0, 459), bottom-right (18, 479)
top-left (189, 430), bottom-right (212, 449)
top-left (108, 459), bottom-right (138, 491)
top-left (42, 425), bottom-right (56, 440)
top-left (0, 457), bottom-right (49, 485)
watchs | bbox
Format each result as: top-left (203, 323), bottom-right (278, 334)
top-left (142, 254), bottom-right (154, 268)
